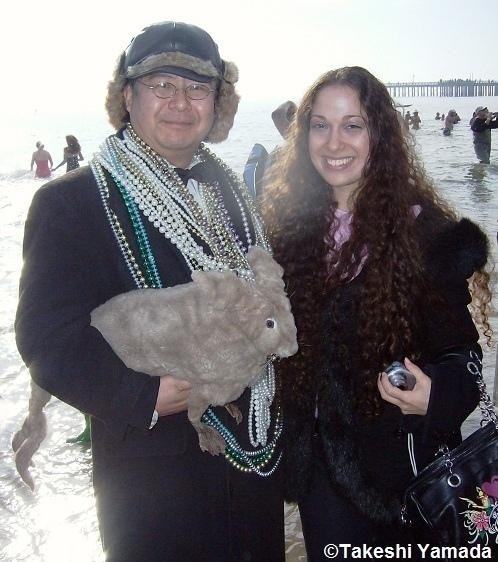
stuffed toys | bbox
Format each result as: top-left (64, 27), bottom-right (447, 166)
top-left (11, 243), bottom-right (301, 492)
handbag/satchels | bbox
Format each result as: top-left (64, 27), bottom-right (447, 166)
top-left (404, 346), bottom-right (498, 561)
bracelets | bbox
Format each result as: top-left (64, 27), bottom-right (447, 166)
top-left (150, 410), bottom-right (159, 430)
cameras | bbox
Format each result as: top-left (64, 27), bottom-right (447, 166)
top-left (378, 361), bottom-right (416, 392)
top-left (492, 113), bottom-right (496, 118)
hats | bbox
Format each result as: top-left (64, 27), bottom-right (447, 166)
top-left (475, 106), bottom-right (489, 116)
top-left (104, 20), bottom-right (240, 145)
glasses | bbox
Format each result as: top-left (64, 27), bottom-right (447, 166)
top-left (131, 76), bottom-right (217, 102)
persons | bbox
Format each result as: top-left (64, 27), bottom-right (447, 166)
top-left (30, 139), bottom-right (53, 179)
top-left (434, 109), bottom-right (460, 136)
top-left (396, 109), bottom-right (423, 132)
top-left (50, 135), bottom-right (83, 174)
top-left (470, 105), bottom-right (498, 164)
top-left (89, 119), bottom-right (287, 478)
top-left (263, 61), bottom-right (484, 561)
top-left (14, 19), bottom-right (286, 561)
